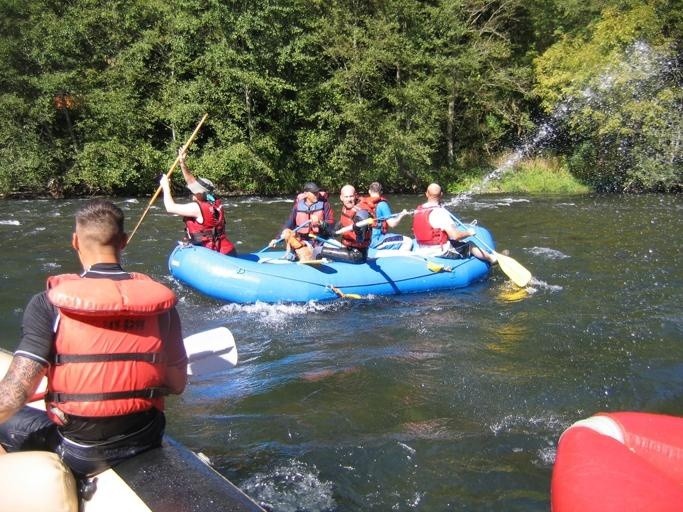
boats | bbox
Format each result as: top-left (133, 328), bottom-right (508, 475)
top-left (167, 223), bottom-right (492, 305)
top-left (547, 410), bottom-right (682, 512)
top-left (0, 347), bottom-right (271, 512)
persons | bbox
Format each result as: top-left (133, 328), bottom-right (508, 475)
top-left (269, 182), bottom-right (335, 262)
top-left (158, 146), bottom-right (237, 260)
top-left (412, 182), bottom-right (511, 265)
top-left (311, 185), bottom-right (373, 264)
top-left (359, 181), bottom-right (414, 252)
top-left (0, 194), bottom-right (188, 484)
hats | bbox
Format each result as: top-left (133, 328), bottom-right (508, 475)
top-left (303, 182), bottom-right (327, 194)
top-left (186, 176), bottom-right (216, 196)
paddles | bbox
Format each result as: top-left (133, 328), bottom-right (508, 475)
top-left (449, 213), bottom-right (532, 288)
top-left (25, 326), bottom-right (242, 406)
